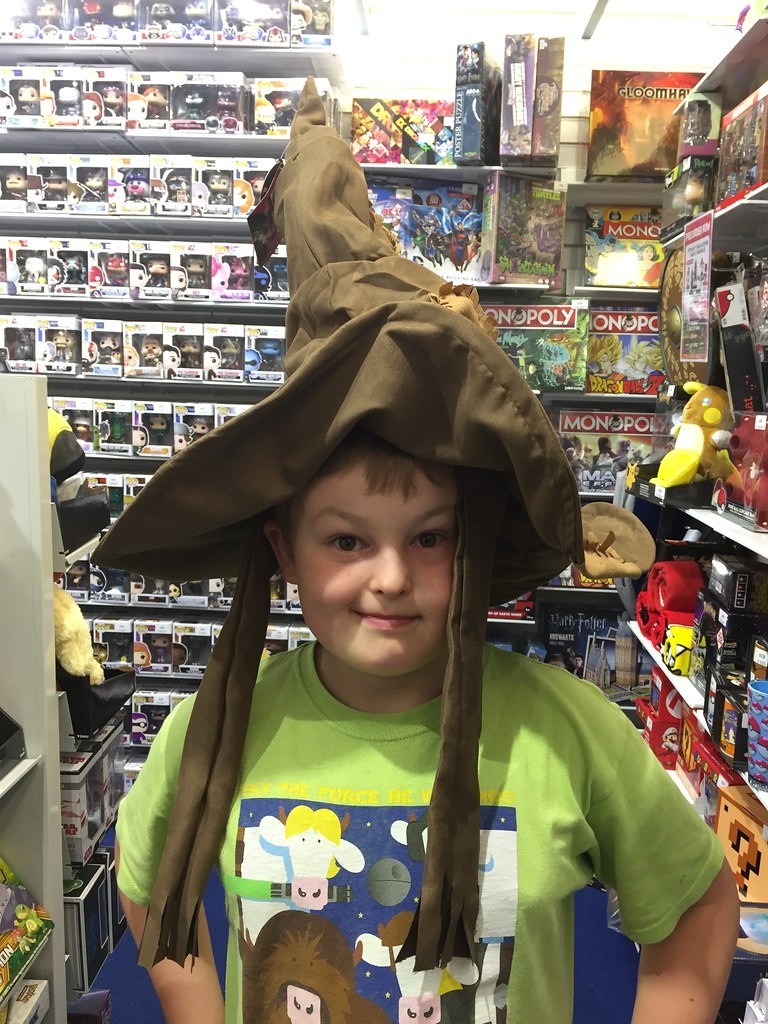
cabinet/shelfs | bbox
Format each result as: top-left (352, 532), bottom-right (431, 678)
top-left (0, 0), bottom-right (768, 1024)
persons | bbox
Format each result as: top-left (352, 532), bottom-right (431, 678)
top-left (587, 438), bottom-right (627, 490)
top-left (92, 302), bottom-right (742, 1024)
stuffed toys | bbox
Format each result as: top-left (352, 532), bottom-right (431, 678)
top-left (53, 583), bottom-right (104, 685)
top-left (649, 382), bottom-right (739, 498)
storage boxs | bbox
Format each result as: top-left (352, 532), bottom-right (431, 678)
top-left (582, 204), bottom-right (668, 289)
top-left (500, 33), bottom-right (532, 168)
top-left (121, 321), bottom-right (162, 380)
top-left (476, 296), bottom-right (588, 391)
top-left (549, 400), bottom-right (669, 492)
top-left (532, 36), bottom-right (566, 168)
top-left (350, 97), bottom-right (457, 166)
top-left (244, 325), bottom-right (287, 386)
top-left (210, 241), bottom-right (252, 304)
top-left (232, 157), bottom-right (274, 219)
top-left (162, 321), bottom-right (203, 380)
top-left (202, 323), bottom-right (244, 382)
top-left (587, 299), bottom-right (670, 395)
top-left (482, 172), bottom-right (567, 290)
top-left (253, 244), bottom-right (291, 305)
top-left (170, 241), bottom-right (211, 302)
top-left (364, 174), bottom-right (481, 282)
top-left (129, 239), bottom-right (170, 300)
top-left (452, 42), bottom-right (501, 167)
top-left (584, 69), bottom-right (708, 183)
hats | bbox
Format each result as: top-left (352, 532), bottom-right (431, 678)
top-left (90, 71), bottom-right (585, 975)
